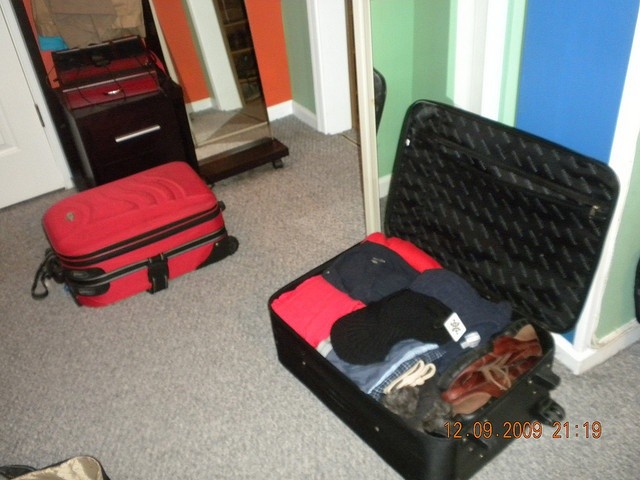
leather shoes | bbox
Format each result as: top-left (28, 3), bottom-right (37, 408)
top-left (436, 318), bottom-right (544, 400)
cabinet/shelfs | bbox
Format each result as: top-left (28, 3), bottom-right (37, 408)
top-left (500, 0), bottom-right (640, 377)
top-left (57, 66), bottom-right (199, 187)
top-left (214, 0), bottom-right (262, 105)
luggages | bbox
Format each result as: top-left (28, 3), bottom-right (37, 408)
top-left (267, 99), bottom-right (620, 480)
top-left (31, 161), bottom-right (238, 307)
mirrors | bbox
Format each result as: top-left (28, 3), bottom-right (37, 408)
top-left (367, 0), bottom-right (458, 236)
